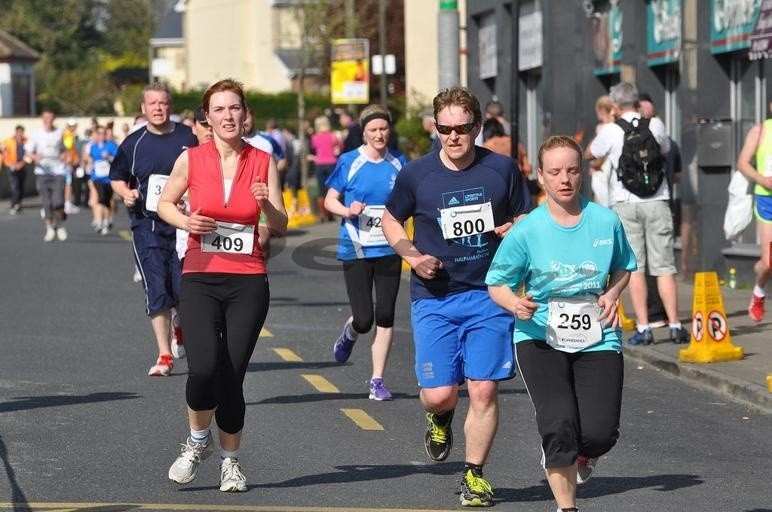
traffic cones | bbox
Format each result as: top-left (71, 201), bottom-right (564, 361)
top-left (606, 273), bottom-right (638, 333)
top-left (400, 215), bottom-right (414, 274)
top-left (679, 271), bottom-right (745, 364)
top-left (766, 373), bottom-right (772, 393)
top-left (282, 188), bottom-right (298, 229)
top-left (296, 187), bottom-right (318, 225)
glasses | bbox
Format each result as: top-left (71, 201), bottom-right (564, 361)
top-left (435, 121), bottom-right (477, 135)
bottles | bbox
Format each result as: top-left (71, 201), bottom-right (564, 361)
top-left (728, 268), bottom-right (736, 289)
top-left (353, 59), bottom-right (366, 82)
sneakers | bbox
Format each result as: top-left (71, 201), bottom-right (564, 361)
top-left (423, 408), bottom-right (455, 463)
top-left (748, 286), bottom-right (767, 322)
top-left (576, 452), bottom-right (600, 487)
top-left (627, 327), bottom-right (655, 347)
top-left (43, 227), bottom-right (55, 242)
top-left (368, 377), bottom-right (393, 402)
top-left (167, 429), bottom-right (213, 486)
top-left (217, 455), bottom-right (248, 493)
top-left (148, 354), bottom-right (174, 377)
top-left (333, 315), bottom-right (359, 363)
top-left (65, 201), bottom-right (114, 237)
top-left (170, 312), bottom-right (187, 360)
top-left (455, 467), bottom-right (496, 507)
top-left (57, 227), bottom-right (67, 242)
top-left (669, 325), bottom-right (691, 345)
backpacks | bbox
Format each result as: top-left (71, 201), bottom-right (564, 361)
top-left (614, 116), bottom-right (666, 198)
